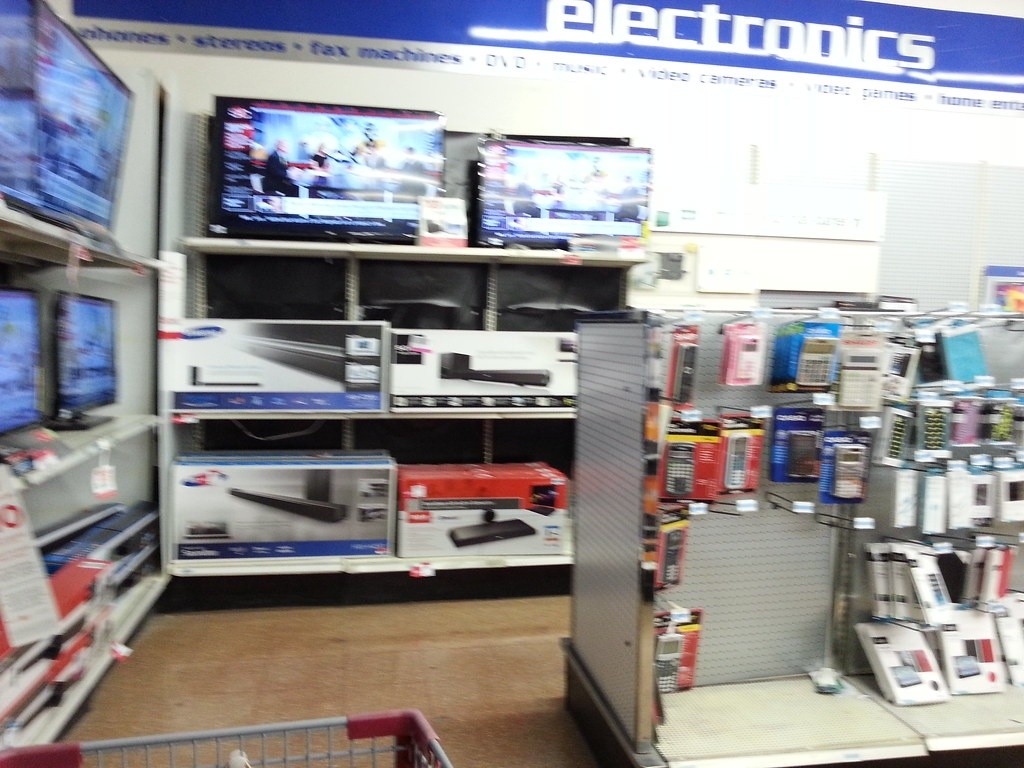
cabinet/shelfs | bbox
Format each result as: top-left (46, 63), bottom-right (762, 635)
top-left (0, 203), bottom-right (171, 752)
top-left (162, 231), bottom-right (644, 577)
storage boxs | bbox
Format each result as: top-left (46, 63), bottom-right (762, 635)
top-left (391, 329), bottom-right (580, 412)
top-left (173, 460), bottom-right (398, 559)
top-left (168, 317), bottom-right (390, 414)
top-left (400, 462), bottom-right (572, 557)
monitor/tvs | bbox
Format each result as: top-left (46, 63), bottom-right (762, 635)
top-left (207, 95), bottom-right (445, 246)
top-left (0, 0), bottom-right (136, 463)
top-left (475, 137), bottom-right (653, 247)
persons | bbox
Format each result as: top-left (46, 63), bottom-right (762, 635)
top-left (261, 140), bottom-right (299, 197)
top-left (402, 145), bottom-right (426, 196)
top-left (513, 175), bottom-right (541, 218)
top-left (614, 176), bottom-right (638, 221)
top-left (309, 145), bottom-right (329, 171)
top-left (365, 140), bottom-right (384, 168)
